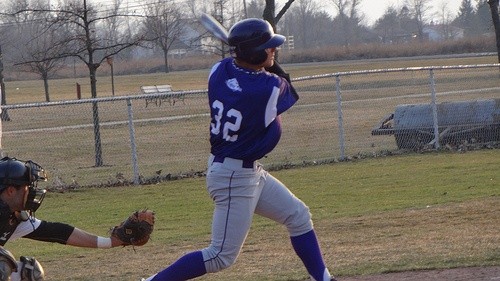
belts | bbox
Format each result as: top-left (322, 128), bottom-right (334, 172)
top-left (213, 156), bottom-right (252, 167)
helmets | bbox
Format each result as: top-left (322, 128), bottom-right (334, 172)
top-left (0, 158), bottom-right (27, 192)
top-left (228, 18), bottom-right (286, 64)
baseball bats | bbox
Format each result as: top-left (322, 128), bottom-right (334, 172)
top-left (198, 12), bottom-right (232, 47)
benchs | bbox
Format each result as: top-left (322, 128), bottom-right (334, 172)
top-left (138, 83), bottom-right (187, 106)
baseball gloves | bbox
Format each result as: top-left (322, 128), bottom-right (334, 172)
top-left (111, 209), bottom-right (155, 246)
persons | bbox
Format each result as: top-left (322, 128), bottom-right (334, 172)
top-left (141, 17), bottom-right (336, 281)
top-left (0, 156), bottom-right (156, 281)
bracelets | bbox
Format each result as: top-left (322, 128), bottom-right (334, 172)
top-left (98, 236), bottom-right (111, 249)
